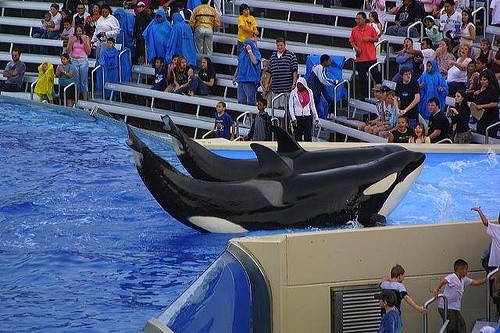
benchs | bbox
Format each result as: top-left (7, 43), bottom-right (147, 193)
top-left (0, 0), bottom-right (500, 144)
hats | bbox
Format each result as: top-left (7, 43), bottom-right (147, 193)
top-left (380, 86), bottom-right (391, 91)
top-left (372, 84), bottom-right (382, 91)
top-left (423, 15), bottom-right (435, 24)
top-left (374, 291), bottom-right (397, 305)
top-left (136, 1), bottom-right (145, 7)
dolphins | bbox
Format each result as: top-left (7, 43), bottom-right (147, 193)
top-left (159, 114), bottom-right (411, 182)
top-left (115, 115), bottom-right (426, 234)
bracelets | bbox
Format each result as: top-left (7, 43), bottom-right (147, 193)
top-left (247, 50), bottom-right (252, 53)
top-left (65, 72), bottom-right (67, 74)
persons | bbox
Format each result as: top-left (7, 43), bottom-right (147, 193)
top-left (145, 0), bottom-right (223, 99)
top-left (358, 0), bottom-right (500, 145)
top-left (374, 291), bottom-right (402, 333)
top-left (34, 63), bottom-right (55, 105)
top-left (306, 54), bottom-right (343, 130)
top-left (56, 54), bottom-right (76, 108)
top-left (378, 265), bottom-right (427, 331)
top-left (471, 207), bottom-right (500, 322)
top-left (233, 38), bottom-right (261, 107)
top-left (65, 26), bottom-right (92, 101)
top-left (237, 3), bottom-right (259, 57)
top-left (34, 0), bottom-right (149, 64)
top-left (0, 50), bottom-right (26, 92)
top-left (212, 102), bottom-right (235, 141)
top-left (97, 37), bottom-right (119, 98)
top-left (267, 38), bottom-right (299, 107)
top-left (260, 60), bottom-right (272, 108)
top-left (350, 12), bottom-right (383, 101)
top-left (433, 259), bottom-right (492, 333)
top-left (245, 99), bottom-right (272, 141)
top-left (289, 77), bottom-right (320, 142)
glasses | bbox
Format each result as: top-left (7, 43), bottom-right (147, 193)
top-left (12, 55), bottom-right (19, 57)
